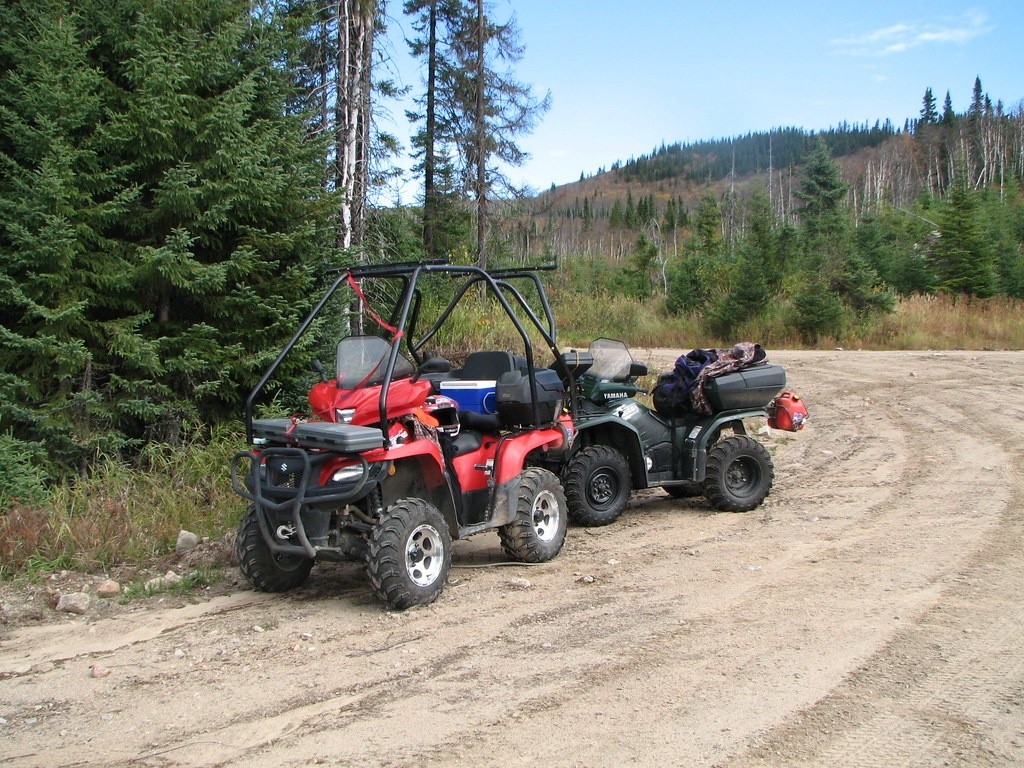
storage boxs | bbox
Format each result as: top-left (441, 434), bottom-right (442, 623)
top-left (440, 381), bottom-right (497, 415)
top-left (549, 352), bottom-right (593, 390)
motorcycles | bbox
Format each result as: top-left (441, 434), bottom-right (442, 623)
top-left (241, 256), bottom-right (577, 614)
top-left (541, 338), bottom-right (807, 533)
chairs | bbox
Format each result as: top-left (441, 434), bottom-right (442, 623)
top-left (442, 352), bottom-right (514, 386)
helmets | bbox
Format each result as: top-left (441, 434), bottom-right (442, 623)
top-left (421, 395), bottom-right (460, 441)
